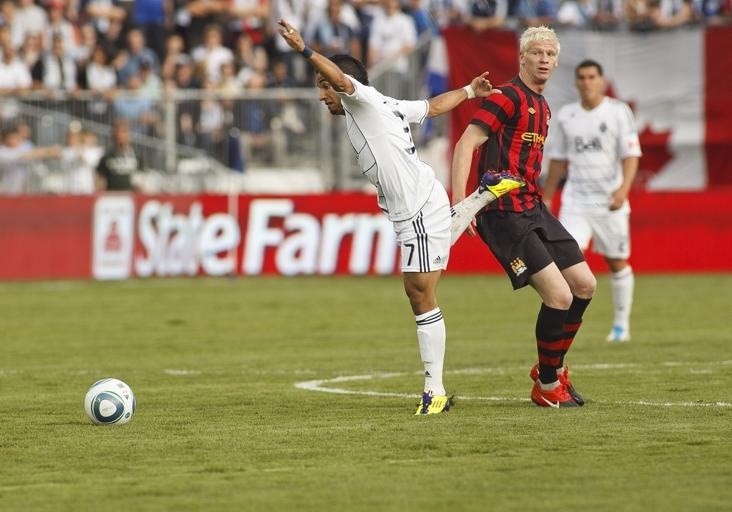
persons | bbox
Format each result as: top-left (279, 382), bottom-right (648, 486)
top-left (451, 26), bottom-right (598, 408)
top-left (279, 18), bottom-right (528, 414)
top-left (453, 0), bottom-right (732, 30)
top-left (1, 1), bottom-right (465, 192)
top-left (542, 59), bottom-right (642, 343)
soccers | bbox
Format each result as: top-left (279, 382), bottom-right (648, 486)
top-left (83, 378), bottom-right (136, 426)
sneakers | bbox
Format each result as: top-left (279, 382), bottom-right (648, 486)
top-left (528, 361), bottom-right (586, 408)
top-left (413, 390), bottom-right (452, 417)
top-left (605, 322), bottom-right (630, 342)
top-left (480, 170), bottom-right (528, 202)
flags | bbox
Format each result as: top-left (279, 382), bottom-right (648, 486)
top-left (442, 22), bottom-right (731, 194)
